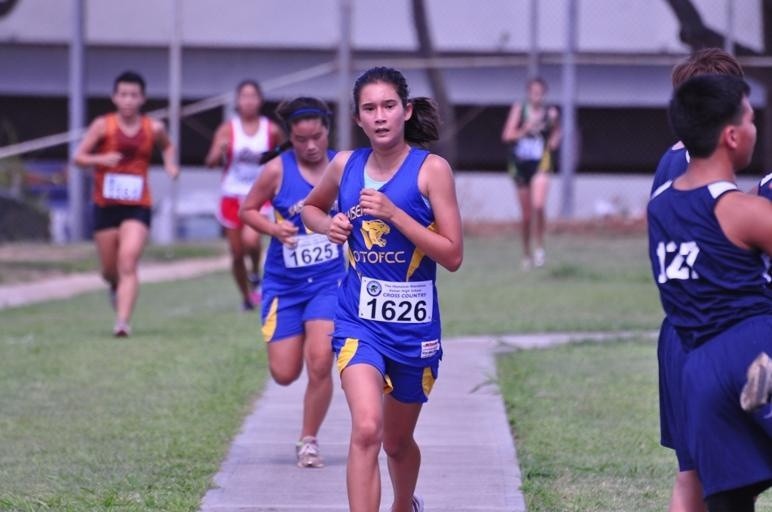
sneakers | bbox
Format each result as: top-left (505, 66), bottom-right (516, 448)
top-left (247, 271), bottom-right (260, 284)
top-left (110, 284), bottom-right (117, 311)
top-left (533, 248), bottom-right (545, 266)
top-left (113, 322), bottom-right (131, 337)
top-left (295, 436), bottom-right (325, 469)
top-left (241, 303), bottom-right (253, 310)
top-left (521, 257), bottom-right (532, 272)
top-left (411, 493), bottom-right (425, 512)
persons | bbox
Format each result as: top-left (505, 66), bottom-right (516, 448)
top-left (655, 46), bottom-right (746, 511)
top-left (501, 77), bottom-right (564, 275)
top-left (298, 65), bottom-right (466, 511)
top-left (72, 71), bottom-right (183, 339)
top-left (204, 79), bottom-right (289, 311)
top-left (644, 69), bottom-right (771, 512)
top-left (237, 96), bottom-right (350, 470)
